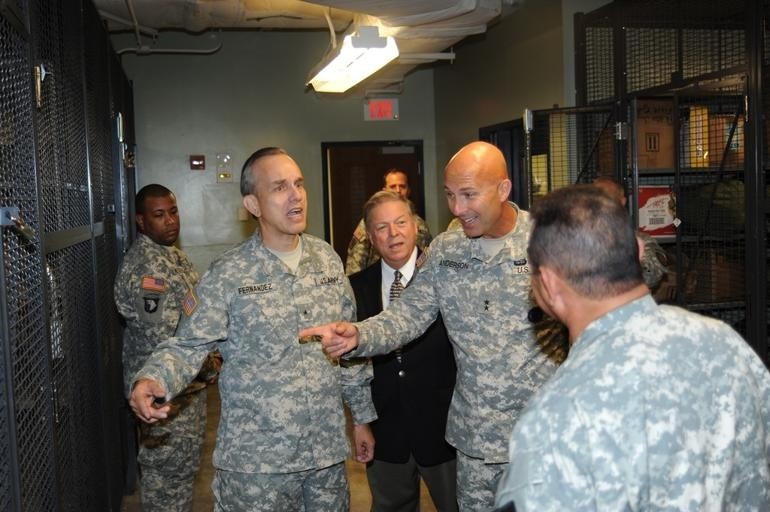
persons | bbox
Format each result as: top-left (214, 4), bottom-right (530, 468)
top-left (509, 186), bottom-right (769, 512)
top-left (347, 188), bottom-right (458, 512)
top-left (346, 165), bottom-right (431, 276)
top-left (115, 183), bottom-right (222, 511)
top-left (298, 142), bottom-right (568, 511)
top-left (128, 145), bottom-right (378, 511)
top-left (591, 175), bottom-right (666, 290)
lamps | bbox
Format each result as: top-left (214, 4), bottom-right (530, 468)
top-left (304, 25), bottom-right (399, 94)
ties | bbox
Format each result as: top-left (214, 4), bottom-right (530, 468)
top-left (389, 271), bottom-right (407, 364)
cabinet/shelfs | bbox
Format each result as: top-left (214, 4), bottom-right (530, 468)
top-left (606, 164), bottom-right (749, 312)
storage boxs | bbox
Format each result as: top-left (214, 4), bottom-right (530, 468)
top-left (599, 90), bottom-right (746, 303)
